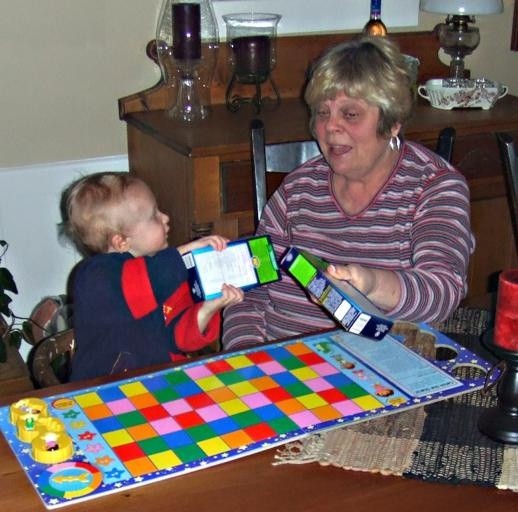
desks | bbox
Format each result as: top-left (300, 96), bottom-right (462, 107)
top-left (0, 294), bottom-right (517, 512)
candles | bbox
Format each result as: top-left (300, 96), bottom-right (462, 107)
top-left (492, 269), bottom-right (518, 353)
top-left (173, 2), bottom-right (199, 60)
top-left (233, 36), bottom-right (272, 82)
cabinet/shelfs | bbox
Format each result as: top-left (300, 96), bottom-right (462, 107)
top-left (120, 31), bottom-right (518, 301)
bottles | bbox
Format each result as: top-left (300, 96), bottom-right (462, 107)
top-left (363, 0), bottom-right (390, 39)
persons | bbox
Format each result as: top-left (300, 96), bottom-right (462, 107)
top-left (55, 171), bottom-right (245, 383)
top-left (220, 37), bottom-right (473, 352)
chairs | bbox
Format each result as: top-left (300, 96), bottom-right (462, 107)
top-left (250, 118), bottom-right (456, 231)
top-left (29, 329), bottom-right (75, 386)
top-left (493, 131), bottom-right (518, 245)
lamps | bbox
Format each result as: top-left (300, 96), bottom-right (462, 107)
top-left (418, 0), bottom-right (503, 112)
